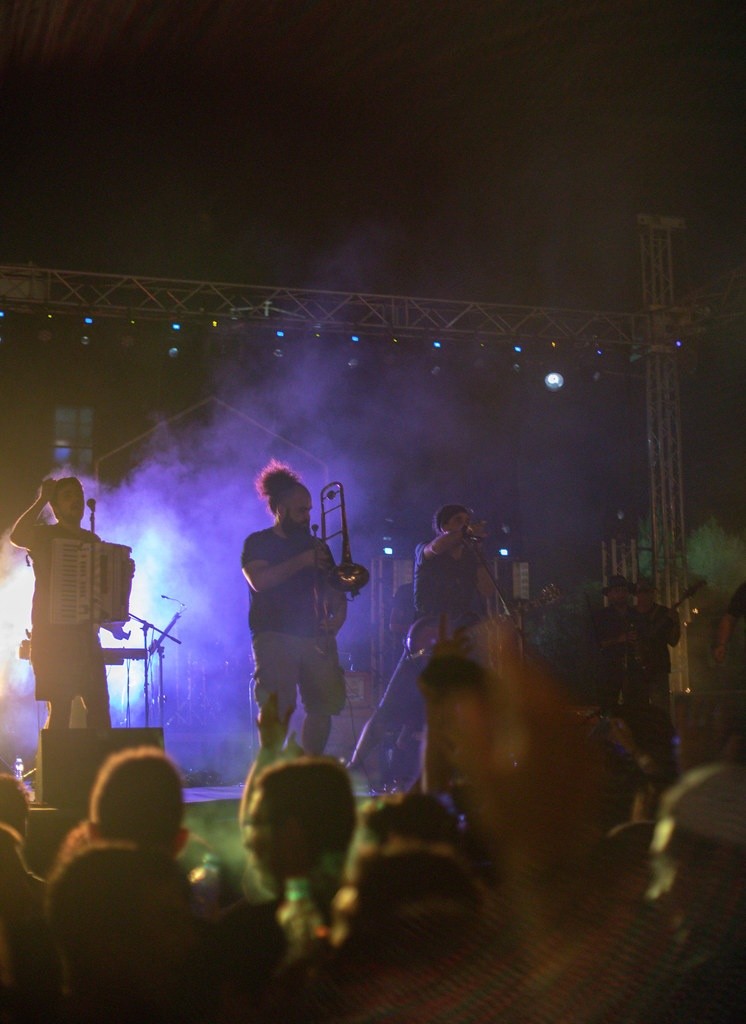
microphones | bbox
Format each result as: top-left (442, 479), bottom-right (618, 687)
top-left (467, 534), bottom-right (481, 542)
top-left (87, 499), bottom-right (96, 512)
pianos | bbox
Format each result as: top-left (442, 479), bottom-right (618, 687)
top-left (20, 639), bottom-right (148, 739)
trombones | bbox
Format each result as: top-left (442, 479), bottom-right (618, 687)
top-left (311, 482), bottom-right (370, 656)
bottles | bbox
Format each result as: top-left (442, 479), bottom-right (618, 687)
top-left (15, 757), bottom-right (23, 778)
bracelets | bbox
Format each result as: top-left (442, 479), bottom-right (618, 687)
top-left (462, 524), bottom-right (481, 544)
top-left (716, 642), bottom-right (727, 650)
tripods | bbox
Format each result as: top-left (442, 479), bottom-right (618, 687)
top-left (167, 628), bottom-right (228, 725)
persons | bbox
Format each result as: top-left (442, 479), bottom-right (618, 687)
top-left (0, 460), bottom-right (745, 1024)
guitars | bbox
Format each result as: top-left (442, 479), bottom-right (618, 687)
top-left (614, 579), bottom-right (708, 673)
top-left (405, 582), bottom-right (566, 679)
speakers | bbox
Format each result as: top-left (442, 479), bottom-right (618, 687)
top-left (34, 727), bottom-right (166, 806)
top-left (326, 703), bottom-right (378, 772)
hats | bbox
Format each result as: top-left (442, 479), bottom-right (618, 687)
top-left (636, 576), bottom-right (656, 597)
top-left (602, 575), bottom-right (633, 597)
top-left (664, 764), bottom-right (746, 845)
